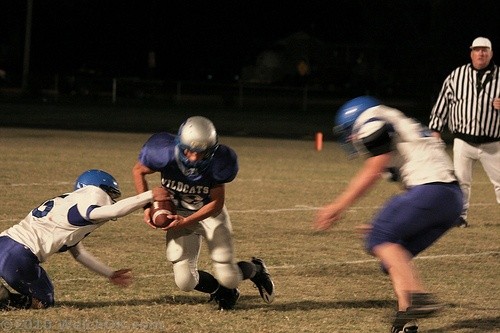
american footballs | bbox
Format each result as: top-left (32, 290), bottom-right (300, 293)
top-left (150, 198), bottom-right (176, 228)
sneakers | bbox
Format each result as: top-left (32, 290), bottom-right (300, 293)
top-left (455, 217), bottom-right (468, 228)
top-left (391, 307), bottom-right (418, 333)
top-left (0, 283), bottom-right (11, 304)
top-left (250, 256), bottom-right (275, 304)
top-left (213, 285), bottom-right (242, 312)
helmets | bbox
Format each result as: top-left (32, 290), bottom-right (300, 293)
top-left (175, 116), bottom-right (220, 181)
top-left (74, 170), bottom-right (121, 199)
top-left (333, 95), bottom-right (383, 135)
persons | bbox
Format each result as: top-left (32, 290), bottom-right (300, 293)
top-left (313, 96), bottom-right (465, 333)
top-left (426, 37), bottom-right (500, 227)
top-left (1, 168), bottom-right (174, 310)
top-left (131, 116), bottom-right (275, 312)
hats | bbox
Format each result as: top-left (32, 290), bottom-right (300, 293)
top-left (469, 37), bottom-right (492, 50)
